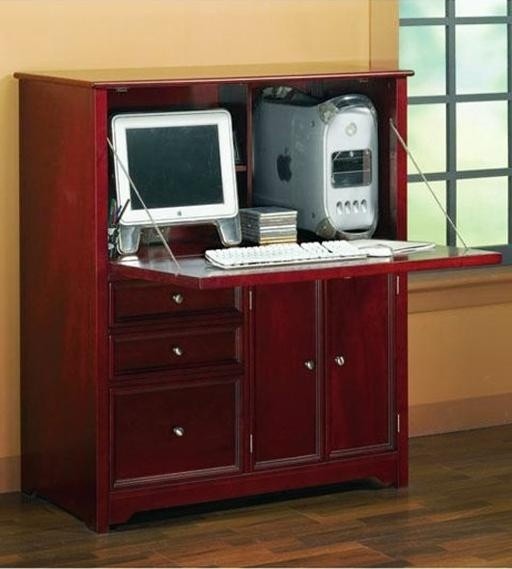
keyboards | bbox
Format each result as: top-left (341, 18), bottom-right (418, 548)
top-left (204, 240), bottom-right (367, 270)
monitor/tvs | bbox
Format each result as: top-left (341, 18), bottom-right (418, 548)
top-left (111, 109), bottom-right (243, 254)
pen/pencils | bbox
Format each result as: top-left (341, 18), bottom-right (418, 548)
top-left (109, 199), bottom-right (131, 226)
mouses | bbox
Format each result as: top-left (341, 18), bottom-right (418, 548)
top-left (359, 245), bottom-right (392, 257)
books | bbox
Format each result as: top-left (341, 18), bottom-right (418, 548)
top-left (239, 206), bottom-right (298, 245)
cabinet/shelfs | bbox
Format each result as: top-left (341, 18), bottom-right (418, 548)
top-left (13, 61), bottom-right (503, 534)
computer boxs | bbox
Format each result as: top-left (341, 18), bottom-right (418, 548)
top-left (253, 85), bottom-right (379, 240)
